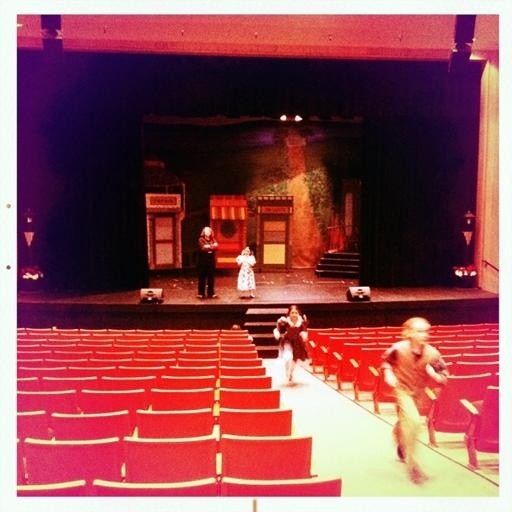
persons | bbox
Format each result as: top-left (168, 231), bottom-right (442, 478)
top-left (378, 315), bottom-right (452, 487)
top-left (272, 313), bottom-right (310, 362)
top-left (195, 225), bottom-right (220, 299)
top-left (235, 245), bottom-right (258, 299)
top-left (272, 304), bottom-right (310, 386)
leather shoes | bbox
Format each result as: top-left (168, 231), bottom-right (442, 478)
top-left (196, 293), bottom-right (256, 299)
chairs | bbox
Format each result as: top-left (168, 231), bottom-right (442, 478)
top-left (16, 324), bottom-right (343, 494)
top-left (303, 320), bottom-right (500, 461)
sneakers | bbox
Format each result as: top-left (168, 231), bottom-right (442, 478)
top-left (392, 424), bottom-right (429, 485)
